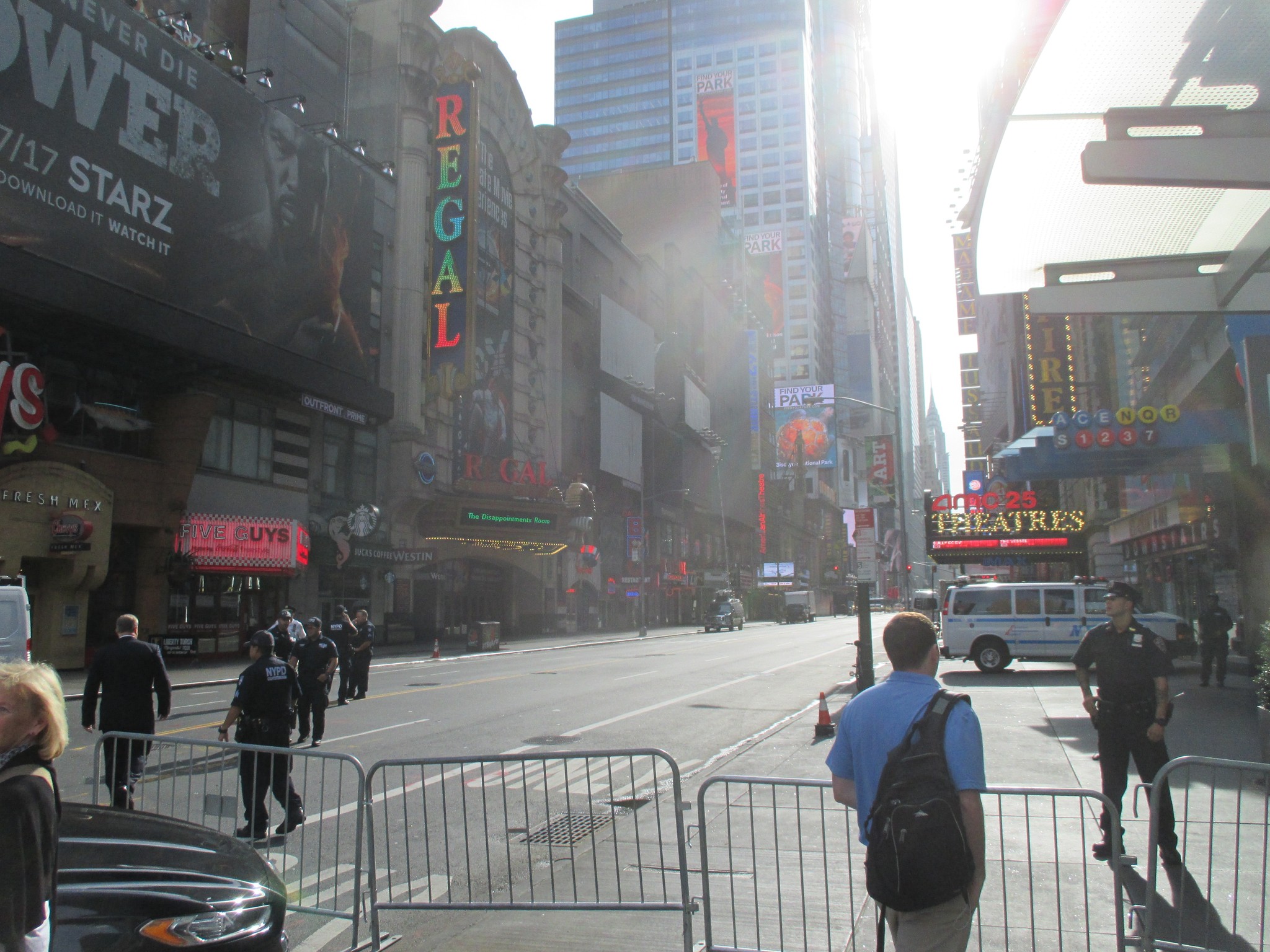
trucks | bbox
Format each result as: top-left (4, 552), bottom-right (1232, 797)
top-left (783, 590), bottom-right (817, 624)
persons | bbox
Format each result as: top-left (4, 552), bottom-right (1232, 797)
top-left (197, 107), bottom-right (371, 371)
top-left (1198, 593), bottom-right (1233, 687)
top-left (218, 629), bottom-right (307, 839)
top-left (268, 605), bottom-right (374, 747)
top-left (697, 102), bottom-right (729, 168)
top-left (0, 659), bottom-right (70, 952)
top-left (1070, 580), bottom-right (1182, 867)
top-left (826, 612), bottom-right (988, 952)
top-left (82, 614), bottom-right (172, 810)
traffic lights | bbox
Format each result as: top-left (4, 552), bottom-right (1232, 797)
top-left (906, 564), bottom-right (912, 573)
top-left (834, 565), bottom-right (839, 573)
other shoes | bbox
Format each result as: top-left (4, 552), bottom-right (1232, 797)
top-left (117, 785), bottom-right (132, 799)
top-left (289, 739), bottom-right (292, 742)
top-left (1217, 681), bottom-right (1224, 686)
top-left (354, 691), bottom-right (366, 700)
top-left (347, 691), bottom-right (356, 697)
top-left (1092, 840), bottom-right (1125, 855)
top-left (1198, 679), bottom-right (1207, 686)
top-left (1160, 848), bottom-right (1182, 864)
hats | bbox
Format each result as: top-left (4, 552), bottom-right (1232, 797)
top-left (303, 617), bottom-right (322, 627)
top-left (244, 630), bottom-right (275, 646)
top-left (334, 605), bottom-right (346, 615)
top-left (1103, 581), bottom-right (1132, 598)
top-left (284, 605), bottom-right (297, 612)
top-left (278, 610), bottom-right (292, 619)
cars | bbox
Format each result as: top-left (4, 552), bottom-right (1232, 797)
top-left (50, 800), bottom-right (289, 952)
top-left (703, 598), bottom-right (746, 631)
top-left (868, 598), bottom-right (885, 609)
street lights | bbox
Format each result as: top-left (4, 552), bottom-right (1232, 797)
top-left (802, 395), bottom-right (912, 613)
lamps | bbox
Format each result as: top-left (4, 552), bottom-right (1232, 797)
top-left (139, 9), bottom-right (397, 178)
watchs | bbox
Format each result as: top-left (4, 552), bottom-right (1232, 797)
top-left (218, 726), bottom-right (228, 733)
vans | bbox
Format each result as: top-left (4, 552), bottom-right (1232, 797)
top-left (911, 590), bottom-right (941, 623)
top-left (937, 576), bottom-right (1195, 674)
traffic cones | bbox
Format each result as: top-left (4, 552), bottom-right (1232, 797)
top-left (431, 639), bottom-right (443, 658)
top-left (814, 691), bottom-right (838, 726)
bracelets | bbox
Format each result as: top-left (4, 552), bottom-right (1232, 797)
top-left (1153, 717), bottom-right (1169, 727)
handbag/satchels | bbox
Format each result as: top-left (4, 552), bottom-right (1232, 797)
top-left (864, 689), bottom-right (976, 912)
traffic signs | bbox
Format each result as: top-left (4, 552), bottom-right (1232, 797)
top-left (854, 528), bottom-right (876, 561)
top-left (855, 560), bottom-right (877, 583)
top-left (852, 508), bottom-right (874, 529)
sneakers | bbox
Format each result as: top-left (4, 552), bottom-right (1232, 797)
top-left (312, 739), bottom-right (321, 746)
top-left (233, 824), bottom-right (266, 838)
top-left (276, 807), bottom-right (307, 834)
top-left (297, 735), bottom-right (309, 743)
top-left (339, 700), bottom-right (350, 706)
